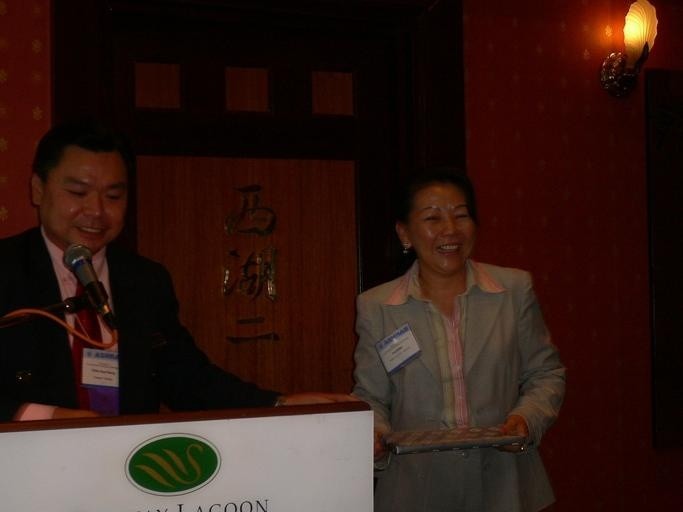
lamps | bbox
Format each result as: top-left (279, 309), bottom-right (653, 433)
top-left (600, 0), bottom-right (658, 98)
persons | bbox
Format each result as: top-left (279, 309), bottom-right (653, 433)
top-left (0, 117), bottom-right (360, 422)
top-left (354, 167), bottom-right (566, 512)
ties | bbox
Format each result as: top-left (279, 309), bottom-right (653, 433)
top-left (70, 280), bottom-right (104, 410)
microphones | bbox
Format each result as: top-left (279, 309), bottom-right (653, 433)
top-left (64, 242), bottom-right (118, 331)
